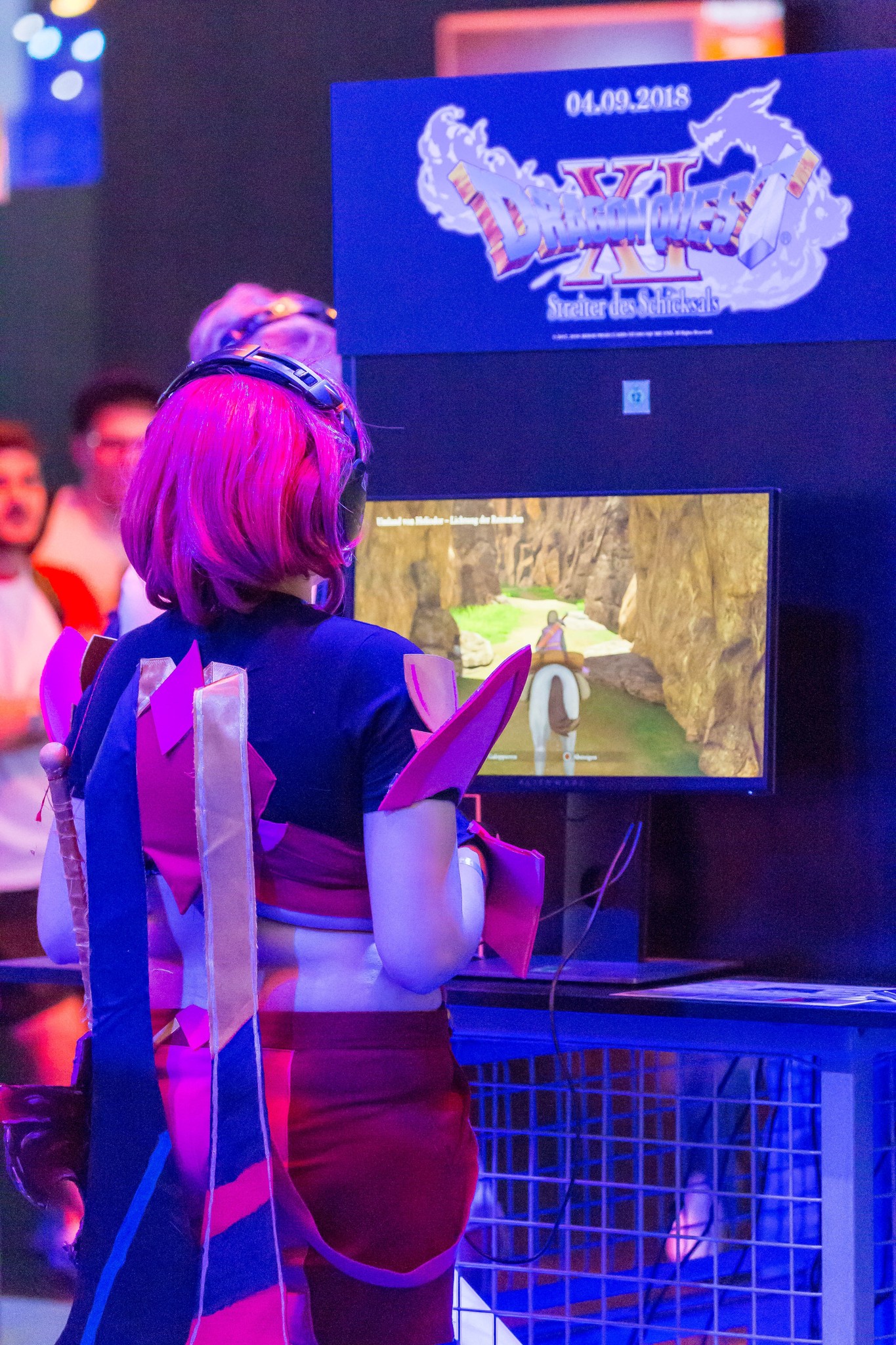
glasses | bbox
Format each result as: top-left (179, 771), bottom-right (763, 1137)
top-left (334, 453), bottom-right (369, 551)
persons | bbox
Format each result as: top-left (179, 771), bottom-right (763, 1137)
top-left (38, 344), bottom-right (550, 1345)
top-left (120, 280), bottom-right (341, 636)
top-left (0, 420), bottom-right (86, 1249)
top-left (32, 376), bottom-right (167, 642)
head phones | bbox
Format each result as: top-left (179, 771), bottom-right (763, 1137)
top-left (152, 343), bottom-right (370, 546)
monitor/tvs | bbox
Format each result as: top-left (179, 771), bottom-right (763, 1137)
top-left (329, 481), bottom-right (777, 989)
